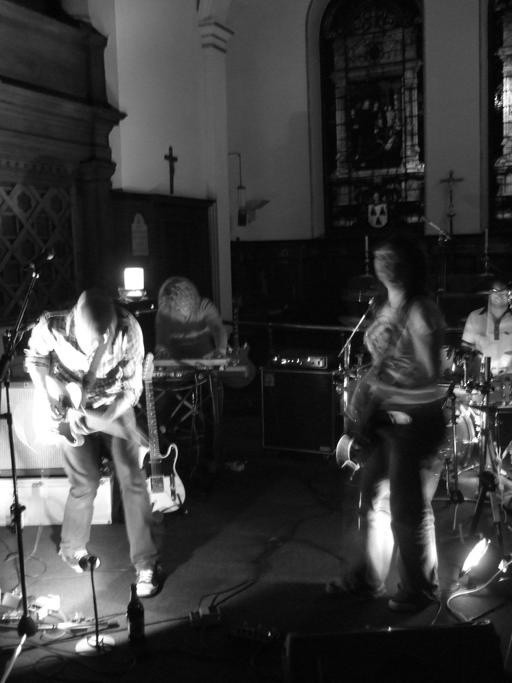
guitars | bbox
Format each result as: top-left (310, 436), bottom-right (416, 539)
top-left (30, 375), bottom-right (151, 448)
top-left (139, 354), bottom-right (186, 515)
top-left (226, 299), bottom-right (257, 387)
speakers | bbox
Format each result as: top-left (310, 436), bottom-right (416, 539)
top-left (259, 365), bottom-right (343, 457)
top-left (285, 622), bottom-right (509, 683)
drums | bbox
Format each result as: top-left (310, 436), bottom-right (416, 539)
top-left (438, 386), bottom-right (486, 473)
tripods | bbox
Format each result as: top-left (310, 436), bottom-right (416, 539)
top-left (1, 304), bottom-right (121, 683)
top-left (431, 388), bottom-right (478, 532)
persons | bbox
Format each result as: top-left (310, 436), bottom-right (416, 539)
top-left (24, 276), bottom-right (176, 594)
top-left (338, 237), bottom-right (453, 623)
top-left (149, 277), bottom-right (227, 367)
top-left (461, 278), bottom-right (511, 473)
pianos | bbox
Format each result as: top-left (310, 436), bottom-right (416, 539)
top-left (139, 356), bottom-right (247, 383)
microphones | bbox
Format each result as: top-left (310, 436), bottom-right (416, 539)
top-left (418, 213), bottom-right (456, 245)
top-left (27, 242), bottom-right (57, 271)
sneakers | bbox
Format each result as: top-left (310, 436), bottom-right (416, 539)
top-left (135, 568), bottom-right (159, 597)
top-left (388, 586), bottom-right (434, 612)
top-left (60, 548), bottom-right (100, 573)
top-left (475, 489), bottom-right (511, 511)
top-left (207, 456), bottom-right (247, 473)
top-left (329, 581), bottom-right (385, 599)
